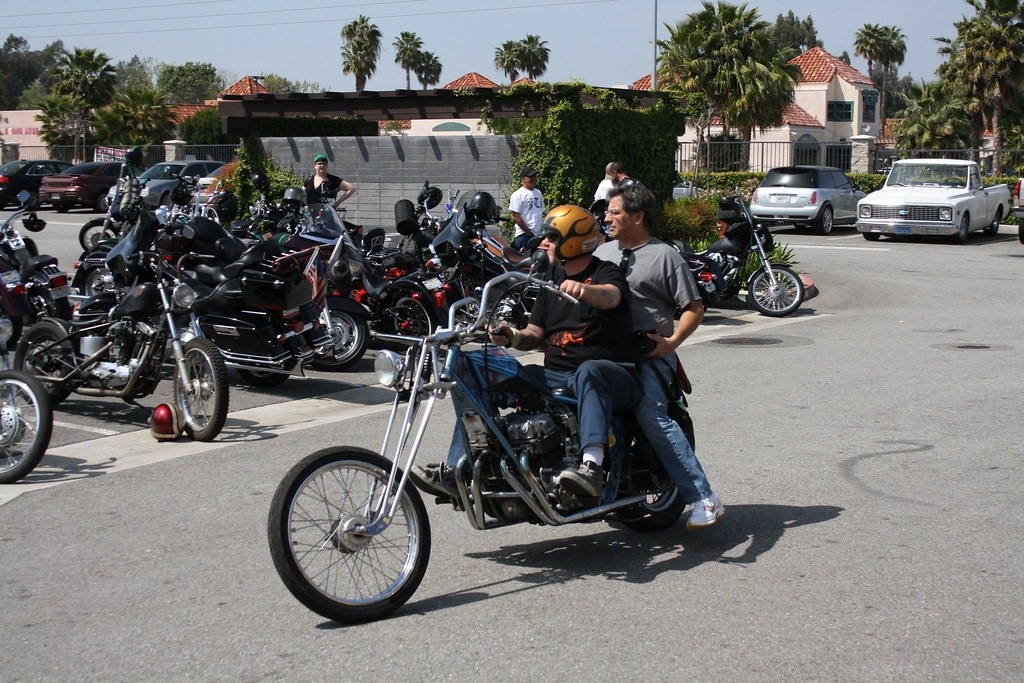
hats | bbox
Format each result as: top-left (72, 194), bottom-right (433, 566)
top-left (520, 167), bottom-right (541, 178)
top-left (314, 153), bottom-right (329, 162)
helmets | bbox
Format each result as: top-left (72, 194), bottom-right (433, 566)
top-left (541, 205), bottom-right (599, 260)
top-left (252, 172), bottom-right (271, 192)
top-left (169, 188), bottom-right (193, 205)
top-left (150, 403), bottom-right (185, 441)
top-left (456, 189), bottom-right (496, 221)
top-left (206, 192), bottom-right (238, 221)
top-left (283, 187), bottom-right (304, 204)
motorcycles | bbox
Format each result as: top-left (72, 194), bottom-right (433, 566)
top-left (659, 185), bottom-right (805, 317)
top-left (0, 157), bottom-right (608, 486)
top-left (267, 190), bottom-right (697, 625)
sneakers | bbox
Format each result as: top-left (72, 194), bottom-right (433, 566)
top-left (409, 463), bottom-right (470, 498)
top-left (561, 460), bottom-right (603, 506)
top-left (687, 493), bottom-right (726, 529)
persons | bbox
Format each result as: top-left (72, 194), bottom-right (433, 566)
top-left (408, 205), bottom-right (640, 506)
top-left (301, 153), bottom-right (355, 215)
top-left (594, 161), bottom-right (656, 237)
top-left (592, 185), bottom-right (725, 529)
top-left (508, 166), bottom-right (545, 249)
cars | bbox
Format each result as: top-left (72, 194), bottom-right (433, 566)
top-left (750, 164), bottom-right (868, 236)
top-left (0, 159), bottom-right (75, 211)
top-left (191, 162), bottom-right (231, 212)
top-left (39, 161), bottom-right (129, 213)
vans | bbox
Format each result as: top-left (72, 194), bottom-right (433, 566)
top-left (105, 159), bottom-right (225, 213)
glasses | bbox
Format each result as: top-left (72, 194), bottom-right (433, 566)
top-left (604, 209), bottom-right (626, 216)
top-left (619, 248), bottom-right (631, 273)
top-left (541, 232), bottom-right (559, 243)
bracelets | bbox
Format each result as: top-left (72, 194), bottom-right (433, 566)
top-left (509, 327), bottom-right (521, 349)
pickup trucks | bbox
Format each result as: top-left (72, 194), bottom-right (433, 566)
top-left (1010, 176), bottom-right (1024, 244)
top-left (856, 158), bottom-right (1011, 244)
